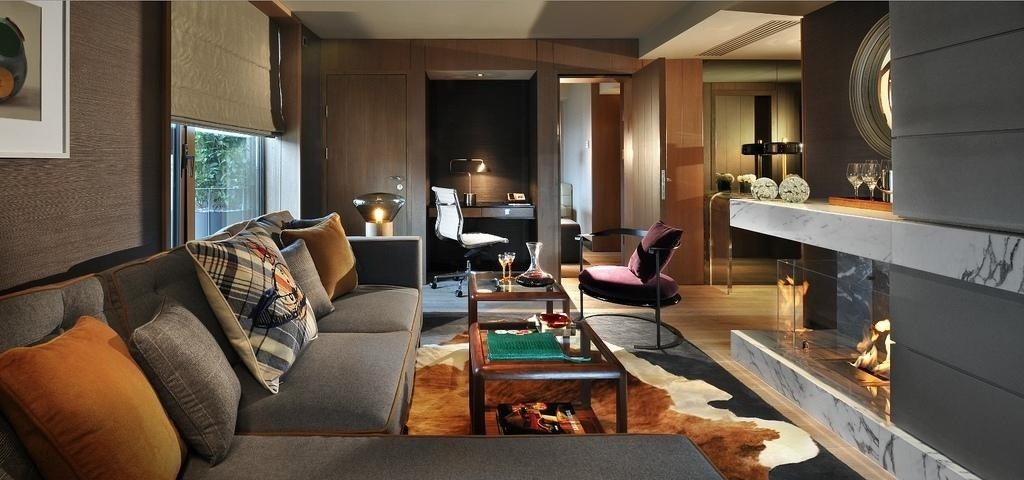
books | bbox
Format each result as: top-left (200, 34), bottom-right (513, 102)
top-left (486, 330), bottom-right (564, 360)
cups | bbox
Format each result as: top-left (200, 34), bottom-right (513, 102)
top-left (463, 192), bottom-right (477, 206)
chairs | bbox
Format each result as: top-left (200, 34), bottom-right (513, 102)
top-left (559, 180), bottom-right (573, 219)
top-left (429, 185), bottom-right (505, 296)
top-left (574, 228), bottom-right (687, 353)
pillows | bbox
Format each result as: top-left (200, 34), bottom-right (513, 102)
top-left (1, 312), bottom-right (193, 479)
top-left (271, 236), bottom-right (336, 322)
top-left (627, 218), bottom-right (682, 281)
top-left (184, 219), bottom-right (320, 397)
top-left (126, 298), bottom-right (243, 464)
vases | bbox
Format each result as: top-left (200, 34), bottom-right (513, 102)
top-left (739, 181), bottom-right (751, 194)
top-left (717, 182), bottom-right (730, 193)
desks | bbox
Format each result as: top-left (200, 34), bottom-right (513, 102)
top-left (428, 202), bottom-right (535, 270)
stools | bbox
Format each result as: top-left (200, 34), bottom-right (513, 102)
top-left (560, 218), bottom-right (583, 263)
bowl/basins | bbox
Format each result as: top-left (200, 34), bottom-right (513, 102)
top-left (540, 312), bottom-right (571, 329)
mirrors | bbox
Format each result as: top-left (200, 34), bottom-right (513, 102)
top-left (703, 58), bottom-right (805, 285)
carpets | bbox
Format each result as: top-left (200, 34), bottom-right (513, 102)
top-left (405, 309), bottom-right (865, 480)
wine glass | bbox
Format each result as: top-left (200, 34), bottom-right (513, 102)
top-left (505, 251), bottom-right (517, 281)
top-left (498, 254), bottom-right (512, 283)
top-left (846, 159), bottom-right (882, 201)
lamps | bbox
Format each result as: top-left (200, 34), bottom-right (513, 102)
top-left (351, 192), bottom-right (406, 237)
top-left (447, 159), bottom-right (491, 205)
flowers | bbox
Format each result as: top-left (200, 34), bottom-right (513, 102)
top-left (714, 171), bottom-right (733, 184)
top-left (736, 173), bottom-right (756, 184)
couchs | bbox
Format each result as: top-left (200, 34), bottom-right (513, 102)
top-left (0, 208), bottom-right (731, 480)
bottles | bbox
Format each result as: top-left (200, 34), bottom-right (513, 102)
top-left (570, 323), bottom-right (578, 336)
top-left (514, 241), bottom-right (557, 288)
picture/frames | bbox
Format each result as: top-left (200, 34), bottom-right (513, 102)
top-left (0, 1), bottom-right (73, 162)
